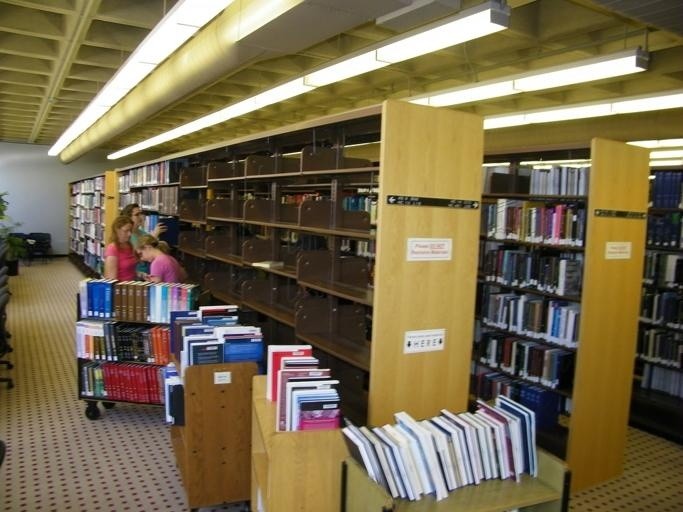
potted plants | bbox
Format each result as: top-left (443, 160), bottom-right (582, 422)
top-left (4, 237), bottom-right (27, 276)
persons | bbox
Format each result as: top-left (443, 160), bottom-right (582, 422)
top-left (120, 202), bottom-right (168, 279)
top-left (133, 235), bottom-right (186, 285)
top-left (103, 216), bottom-right (138, 280)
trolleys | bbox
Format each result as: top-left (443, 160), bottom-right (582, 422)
top-left (75, 291), bottom-right (165, 422)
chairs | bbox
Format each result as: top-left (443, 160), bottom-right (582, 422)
top-left (0, 238), bottom-right (14, 389)
top-left (7, 232), bottom-right (50, 266)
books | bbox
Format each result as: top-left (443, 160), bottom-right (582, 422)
top-left (164, 303), bottom-right (263, 426)
top-left (75, 277), bottom-right (199, 405)
top-left (469, 162), bottom-right (590, 432)
top-left (341, 395), bottom-right (537, 502)
top-left (633, 171), bottom-right (683, 398)
top-left (68, 176), bottom-right (105, 278)
top-left (252, 259), bottom-right (284, 269)
top-left (279, 194), bottom-right (377, 256)
top-left (117, 160), bottom-right (177, 246)
top-left (265, 344), bottom-right (342, 432)
